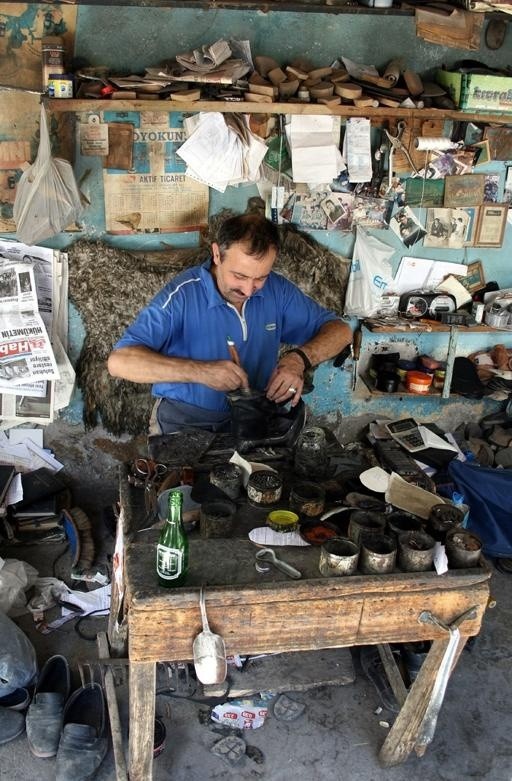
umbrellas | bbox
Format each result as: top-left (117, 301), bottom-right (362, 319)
top-left (359, 641), bottom-right (432, 713)
top-left (0, 655), bottom-right (108, 781)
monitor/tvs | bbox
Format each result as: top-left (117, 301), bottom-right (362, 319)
top-left (1, 465), bottom-right (67, 546)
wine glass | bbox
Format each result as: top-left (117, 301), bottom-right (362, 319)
top-left (289, 388), bottom-right (296, 394)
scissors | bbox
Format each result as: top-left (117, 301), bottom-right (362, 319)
top-left (193, 586), bottom-right (227, 685)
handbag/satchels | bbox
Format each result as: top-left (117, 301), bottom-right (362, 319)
top-left (284, 347), bottom-right (311, 380)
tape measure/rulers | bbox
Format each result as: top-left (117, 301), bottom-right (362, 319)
top-left (384, 121), bottom-right (419, 186)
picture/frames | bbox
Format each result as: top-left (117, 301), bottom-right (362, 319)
top-left (93, 447), bottom-right (491, 781)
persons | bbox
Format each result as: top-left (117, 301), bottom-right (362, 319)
top-left (294, 192), bottom-right (353, 230)
top-left (368, 204), bottom-right (384, 226)
top-left (378, 183), bottom-right (389, 200)
top-left (106, 213), bottom-right (355, 435)
top-left (450, 218), bottom-right (465, 241)
top-left (386, 186), bottom-right (398, 203)
top-left (430, 216), bottom-right (444, 239)
top-left (483, 178), bottom-right (497, 203)
top-left (400, 227), bottom-right (426, 249)
top-left (395, 182), bottom-right (406, 207)
top-left (398, 213), bottom-right (418, 235)
top-left (351, 202), bottom-right (367, 222)
top-left (445, 217), bottom-right (457, 240)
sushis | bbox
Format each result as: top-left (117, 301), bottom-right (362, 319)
top-left (385, 417), bottom-right (430, 453)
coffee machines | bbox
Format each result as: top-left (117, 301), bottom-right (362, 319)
top-left (136, 459), bottom-right (167, 517)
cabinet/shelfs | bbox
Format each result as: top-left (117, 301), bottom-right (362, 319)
top-left (155, 491), bottom-right (189, 589)
